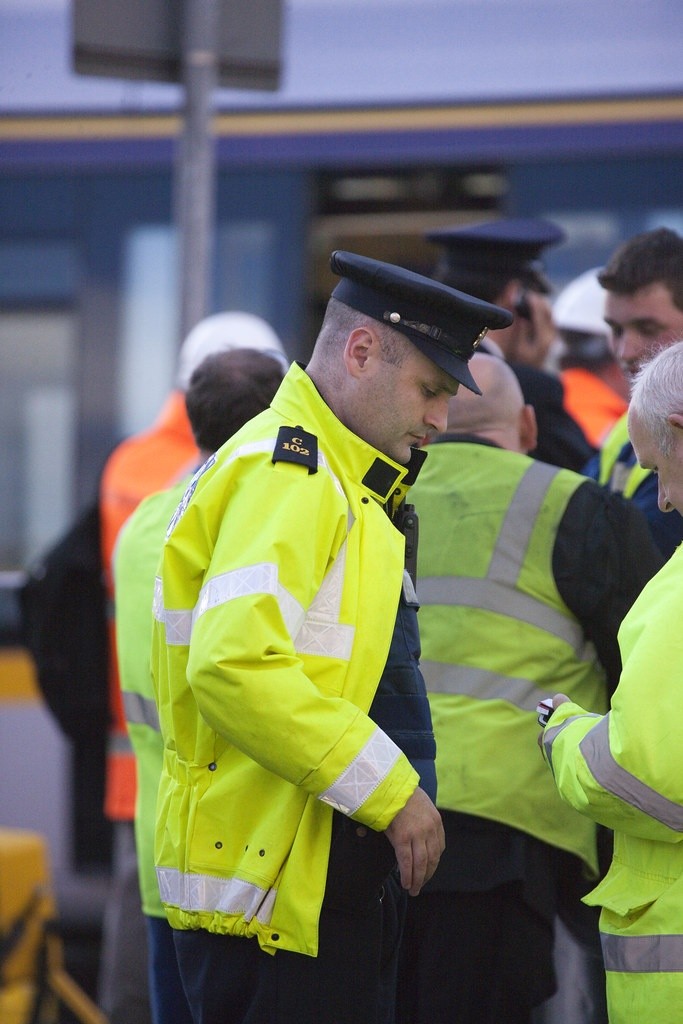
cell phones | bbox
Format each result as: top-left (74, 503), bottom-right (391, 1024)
top-left (516, 287), bottom-right (531, 322)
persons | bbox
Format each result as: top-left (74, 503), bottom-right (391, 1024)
top-left (100, 217), bottom-right (683, 1024)
top-left (150, 250), bottom-right (513, 1024)
top-left (537, 341), bottom-right (683, 1024)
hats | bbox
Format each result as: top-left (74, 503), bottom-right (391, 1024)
top-left (329, 249), bottom-right (512, 397)
top-left (423, 216), bottom-right (567, 302)
top-left (174, 310), bottom-right (288, 392)
